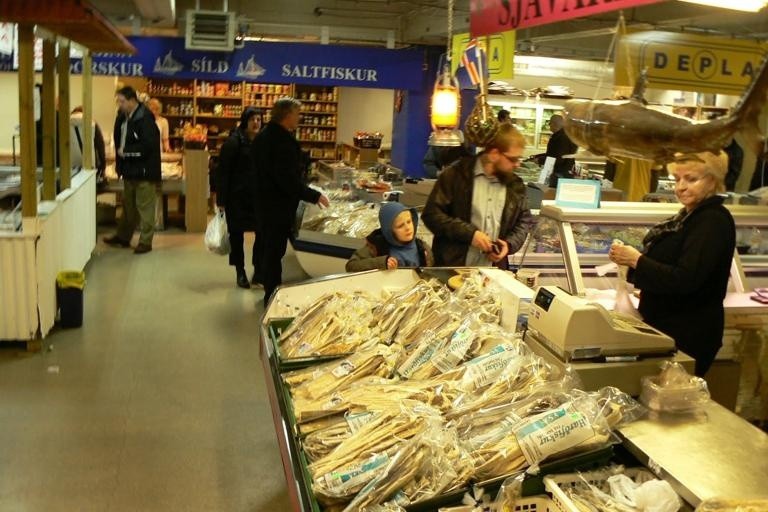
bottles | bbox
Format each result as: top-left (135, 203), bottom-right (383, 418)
top-left (147, 78), bottom-right (336, 159)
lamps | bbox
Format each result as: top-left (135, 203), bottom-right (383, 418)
top-left (426, 0), bottom-right (465, 148)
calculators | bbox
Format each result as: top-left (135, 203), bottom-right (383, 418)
top-left (754, 288), bottom-right (768, 299)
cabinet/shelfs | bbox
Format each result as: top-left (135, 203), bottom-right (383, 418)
top-left (507, 199), bottom-right (768, 297)
top-left (182, 149), bottom-right (208, 234)
top-left (486, 89), bottom-right (575, 150)
top-left (288, 162), bottom-right (434, 278)
top-left (148, 77), bottom-right (340, 161)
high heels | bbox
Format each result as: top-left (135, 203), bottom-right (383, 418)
top-left (236, 269), bottom-right (250, 288)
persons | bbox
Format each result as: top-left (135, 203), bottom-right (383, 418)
top-left (421, 123), bottom-right (533, 269)
top-left (533, 114), bottom-right (578, 187)
top-left (36, 86), bottom-right (170, 254)
top-left (498, 110), bottom-right (510, 121)
top-left (723, 136), bottom-right (744, 193)
top-left (608, 149), bottom-right (735, 372)
top-left (345, 201), bottom-right (434, 272)
top-left (248, 94), bottom-right (329, 305)
top-left (216, 105), bottom-right (264, 289)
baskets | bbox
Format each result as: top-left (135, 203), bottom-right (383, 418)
top-left (354, 139), bottom-right (381, 149)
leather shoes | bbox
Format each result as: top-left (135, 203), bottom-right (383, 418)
top-left (134, 243), bottom-right (152, 253)
top-left (103, 235), bottom-right (129, 247)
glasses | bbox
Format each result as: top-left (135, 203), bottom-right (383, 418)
top-left (501, 151), bottom-right (519, 162)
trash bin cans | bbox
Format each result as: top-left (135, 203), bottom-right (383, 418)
top-left (56, 271), bottom-right (86, 329)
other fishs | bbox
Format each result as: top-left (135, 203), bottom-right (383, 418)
top-left (561, 51), bottom-right (768, 166)
